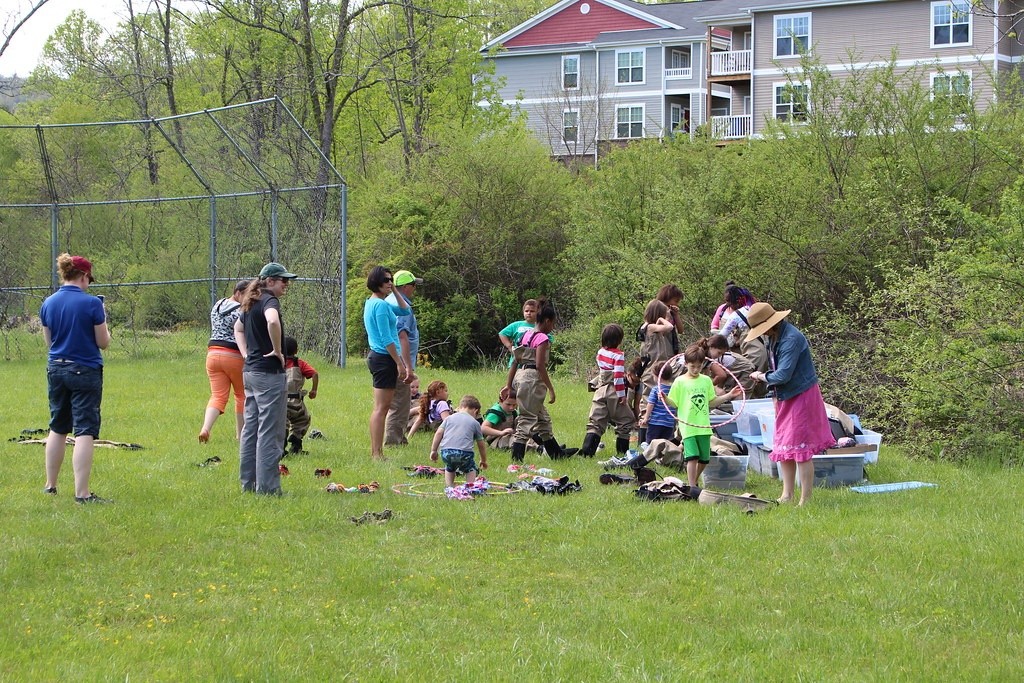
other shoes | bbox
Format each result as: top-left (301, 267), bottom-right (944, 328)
top-left (198, 431), bottom-right (209, 444)
top-left (772, 497), bottom-right (780, 505)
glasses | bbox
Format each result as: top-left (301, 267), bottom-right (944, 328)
top-left (401, 282), bottom-right (416, 287)
top-left (273, 277), bottom-right (288, 285)
top-left (383, 277), bottom-right (393, 283)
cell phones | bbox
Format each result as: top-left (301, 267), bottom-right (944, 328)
top-left (97, 295), bottom-right (104, 303)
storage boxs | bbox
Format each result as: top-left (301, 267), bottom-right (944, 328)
top-left (732, 397), bottom-right (882, 487)
top-left (700, 454), bottom-right (750, 490)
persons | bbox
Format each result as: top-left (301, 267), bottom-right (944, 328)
top-left (383, 270), bottom-right (423, 447)
top-left (499, 297), bottom-right (580, 464)
top-left (198, 280), bottom-right (251, 444)
top-left (577, 281), bottom-right (772, 501)
top-left (234, 263), bottom-right (298, 500)
top-left (41, 254), bottom-right (114, 507)
top-left (363, 266), bottom-right (411, 465)
top-left (744, 302), bottom-right (837, 507)
top-left (499, 299), bottom-right (553, 369)
top-left (481, 386), bottom-right (544, 456)
top-left (281, 337), bottom-right (318, 461)
top-left (403, 373), bottom-right (426, 441)
top-left (429, 395), bottom-right (488, 488)
top-left (420, 380), bottom-right (458, 432)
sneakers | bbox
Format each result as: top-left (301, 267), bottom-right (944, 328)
top-left (74, 491), bottom-right (114, 507)
top-left (42, 487), bottom-right (57, 496)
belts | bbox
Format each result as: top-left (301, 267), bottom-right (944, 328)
top-left (52, 358), bottom-right (75, 363)
top-left (287, 394), bottom-right (303, 400)
top-left (520, 365), bottom-right (536, 369)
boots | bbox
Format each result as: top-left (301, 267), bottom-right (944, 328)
top-left (512, 441), bottom-right (525, 464)
top-left (540, 436), bottom-right (580, 460)
top-left (282, 429), bottom-right (289, 457)
top-left (581, 432), bottom-right (600, 460)
top-left (532, 432), bottom-right (566, 450)
top-left (616, 437), bottom-right (629, 455)
top-left (288, 436), bottom-right (309, 455)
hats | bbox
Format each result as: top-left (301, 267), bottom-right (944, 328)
top-left (743, 302), bottom-right (791, 343)
top-left (258, 262), bottom-right (298, 280)
top-left (71, 256), bottom-right (94, 283)
top-left (392, 270), bottom-right (423, 287)
